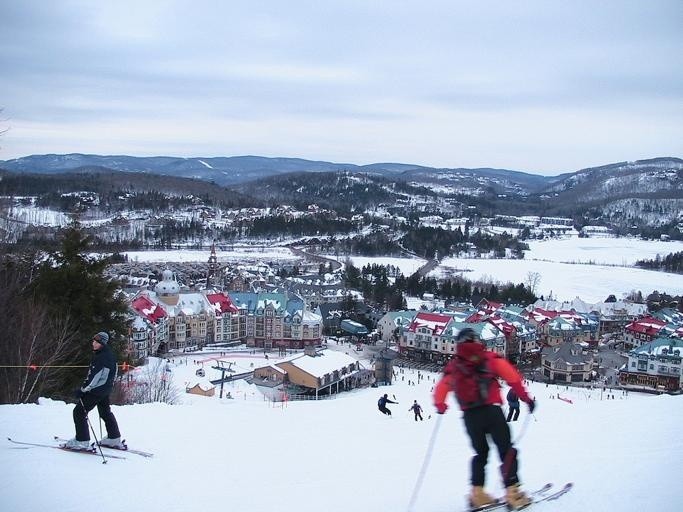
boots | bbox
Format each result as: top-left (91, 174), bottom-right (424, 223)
top-left (506, 485), bottom-right (529, 509)
top-left (470, 486), bottom-right (498, 509)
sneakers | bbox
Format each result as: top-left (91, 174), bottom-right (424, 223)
top-left (99, 436), bottom-right (122, 446)
top-left (63, 439), bottom-right (89, 450)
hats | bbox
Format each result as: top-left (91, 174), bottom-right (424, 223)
top-left (457, 328), bottom-right (480, 343)
top-left (93, 331), bottom-right (109, 346)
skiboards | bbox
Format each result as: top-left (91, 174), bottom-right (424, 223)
top-left (465, 482), bottom-right (572, 512)
top-left (8, 436), bottom-right (154, 459)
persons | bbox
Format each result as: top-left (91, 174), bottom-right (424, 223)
top-left (377, 393), bottom-right (399, 416)
top-left (504, 387), bottom-right (520, 422)
top-left (408, 399), bottom-right (424, 421)
top-left (434, 328), bottom-right (535, 510)
top-left (64, 332), bottom-right (121, 448)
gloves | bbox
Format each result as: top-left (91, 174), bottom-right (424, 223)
top-left (72, 388), bottom-right (83, 399)
